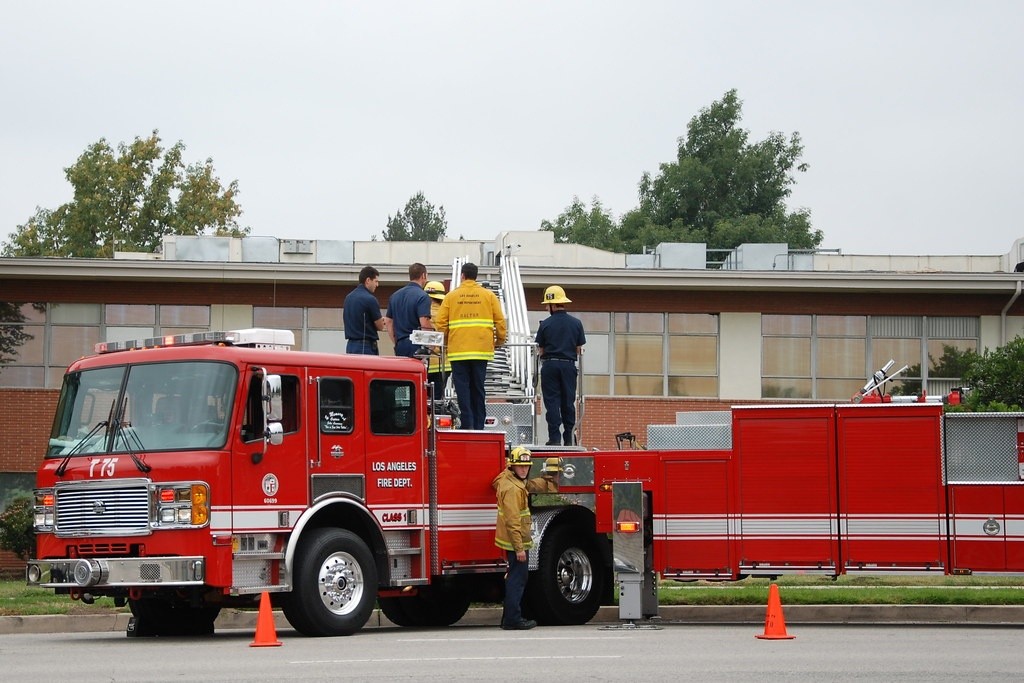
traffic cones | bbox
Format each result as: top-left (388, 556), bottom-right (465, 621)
top-left (757, 584), bottom-right (795, 640)
top-left (248, 591), bottom-right (282, 645)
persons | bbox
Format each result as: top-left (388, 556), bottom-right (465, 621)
top-left (384, 263), bottom-right (432, 361)
top-left (527, 457), bottom-right (564, 493)
top-left (535, 285), bottom-right (587, 446)
top-left (492, 447), bottom-right (548, 630)
top-left (422, 281), bottom-right (452, 400)
top-left (342, 267), bottom-right (386, 356)
top-left (435, 262), bottom-right (507, 430)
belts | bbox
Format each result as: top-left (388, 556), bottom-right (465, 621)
top-left (547, 358), bottom-right (575, 363)
top-left (403, 337), bottom-right (410, 340)
top-left (350, 338), bottom-right (372, 343)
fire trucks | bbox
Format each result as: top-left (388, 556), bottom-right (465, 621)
top-left (24, 340), bottom-right (1024, 638)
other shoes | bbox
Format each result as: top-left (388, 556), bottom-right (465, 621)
top-left (565, 442), bottom-right (576, 446)
top-left (503, 619), bottom-right (537, 630)
top-left (500, 618), bottom-right (527, 628)
top-left (545, 440), bottom-right (561, 446)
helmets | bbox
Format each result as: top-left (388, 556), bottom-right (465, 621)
top-left (424, 281), bottom-right (446, 299)
top-left (541, 286), bottom-right (572, 304)
top-left (507, 447), bottom-right (533, 465)
top-left (540, 458), bottom-right (564, 471)
